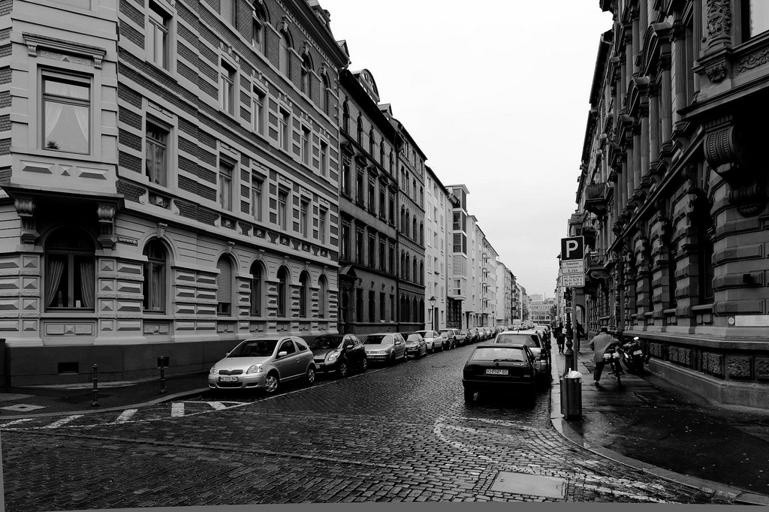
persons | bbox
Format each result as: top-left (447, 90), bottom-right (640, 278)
top-left (553, 319), bottom-right (585, 354)
top-left (590, 325), bottom-right (621, 386)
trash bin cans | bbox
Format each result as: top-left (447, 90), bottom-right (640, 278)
top-left (560, 368), bottom-right (582, 424)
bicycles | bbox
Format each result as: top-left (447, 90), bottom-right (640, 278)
top-left (607, 349), bottom-right (625, 383)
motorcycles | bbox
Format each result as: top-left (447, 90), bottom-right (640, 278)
top-left (618, 337), bottom-right (650, 378)
